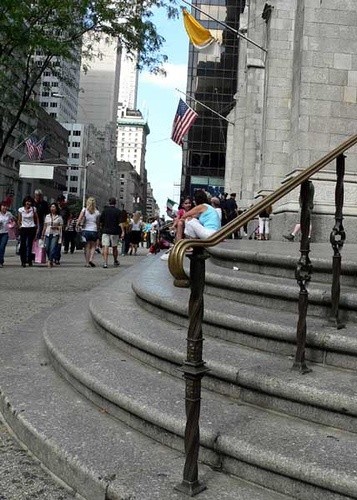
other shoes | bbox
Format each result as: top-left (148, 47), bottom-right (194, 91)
top-left (0, 263), bottom-right (3, 268)
top-left (88, 261), bottom-right (96, 267)
top-left (113, 260), bottom-right (120, 266)
top-left (122, 253), bottom-right (138, 256)
top-left (103, 264), bottom-right (107, 268)
top-left (64, 251), bottom-right (68, 254)
top-left (28, 261), bottom-right (33, 266)
top-left (22, 263), bottom-right (26, 268)
top-left (47, 261), bottom-right (53, 269)
top-left (70, 251), bottom-right (74, 254)
top-left (84, 265), bottom-right (89, 267)
top-left (282, 233), bottom-right (294, 242)
top-left (299, 237), bottom-right (312, 243)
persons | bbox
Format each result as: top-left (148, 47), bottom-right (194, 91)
top-left (160, 190), bottom-right (221, 260)
top-left (41, 202), bottom-right (63, 268)
top-left (0, 200), bottom-right (17, 268)
top-left (61, 192), bottom-right (240, 255)
top-left (77, 197), bottom-right (101, 268)
top-left (257, 195), bottom-right (273, 240)
top-left (100, 198), bottom-right (125, 268)
top-left (283, 196), bottom-right (314, 242)
top-left (18, 196), bottom-right (39, 268)
top-left (31, 190), bottom-right (49, 240)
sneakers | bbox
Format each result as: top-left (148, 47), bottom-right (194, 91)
top-left (160, 253), bottom-right (169, 261)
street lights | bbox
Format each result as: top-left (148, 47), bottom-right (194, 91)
top-left (83, 160), bottom-right (95, 210)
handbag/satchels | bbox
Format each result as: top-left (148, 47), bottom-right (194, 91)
top-left (33, 207), bottom-right (37, 225)
top-left (32, 240), bottom-right (46, 263)
top-left (79, 208), bottom-right (85, 228)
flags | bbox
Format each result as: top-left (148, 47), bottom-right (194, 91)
top-left (171, 99), bottom-right (199, 146)
top-left (26, 133), bottom-right (37, 159)
top-left (36, 138), bottom-right (45, 160)
top-left (167, 199), bottom-right (176, 212)
top-left (181, 6), bottom-right (217, 49)
